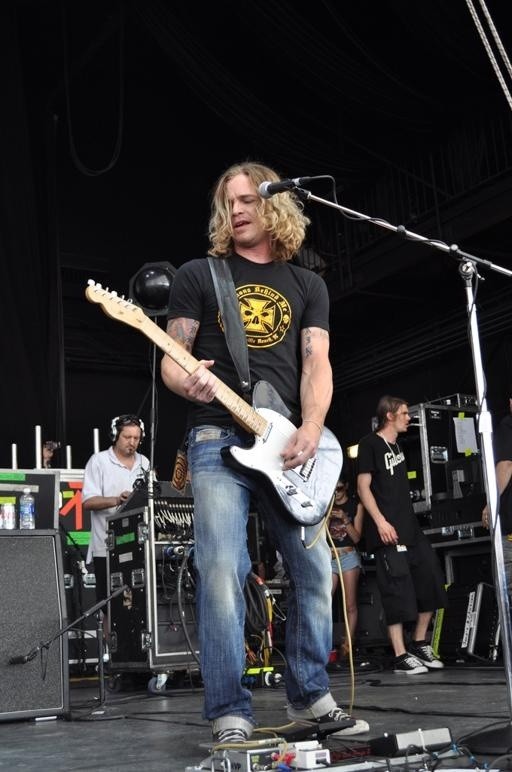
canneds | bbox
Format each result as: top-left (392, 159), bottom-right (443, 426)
top-left (1, 503), bottom-right (16, 530)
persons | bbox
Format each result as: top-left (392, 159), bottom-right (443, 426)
top-left (160, 162), bottom-right (370, 744)
top-left (354, 396), bottom-right (444, 675)
top-left (82, 416), bottom-right (158, 616)
top-left (482, 397), bottom-right (511, 602)
top-left (330, 479), bottom-right (364, 656)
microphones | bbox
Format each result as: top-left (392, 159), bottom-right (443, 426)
top-left (260, 176), bottom-right (298, 197)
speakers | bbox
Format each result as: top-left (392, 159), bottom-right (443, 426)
top-left (2, 530), bottom-right (70, 725)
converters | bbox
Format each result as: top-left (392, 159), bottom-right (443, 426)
top-left (280, 743), bottom-right (333, 768)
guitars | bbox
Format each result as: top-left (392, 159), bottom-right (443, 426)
top-left (85, 277), bottom-right (346, 525)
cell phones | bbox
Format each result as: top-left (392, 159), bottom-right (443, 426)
top-left (330, 505), bottom-right (338, 516)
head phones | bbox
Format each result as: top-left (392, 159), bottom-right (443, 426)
top-left (107, 414), bottom-right (147, 441)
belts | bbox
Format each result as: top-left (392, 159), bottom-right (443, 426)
top-left (331, 546), bottom-right (353, 558)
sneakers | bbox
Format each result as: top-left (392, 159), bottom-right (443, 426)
top-left (287, 707), bottom-right (370, 737)
top-left (391, 652), bottom-right (429, 675)
top-left (211, 727), bottom-right (248, 746)
top-left (407, 639), bottom-right (444, 668)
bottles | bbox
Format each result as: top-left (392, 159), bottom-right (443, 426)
top-left (19, 488), bottom-right (36, 530)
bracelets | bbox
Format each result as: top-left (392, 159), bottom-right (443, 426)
top-left (303, 419), bottom-right (322, 435)
